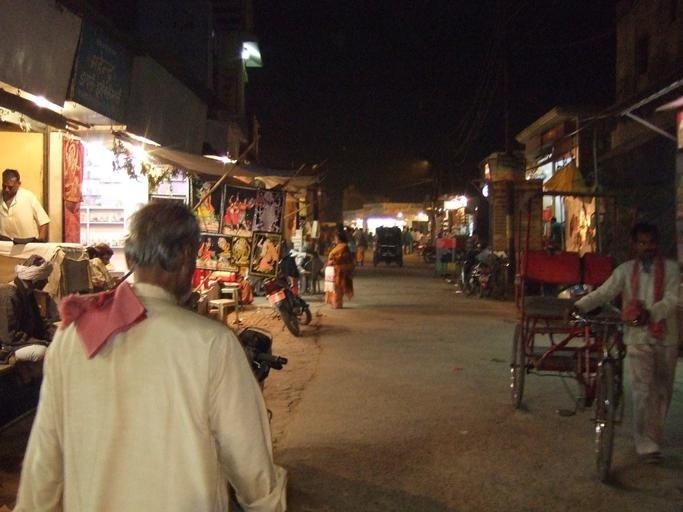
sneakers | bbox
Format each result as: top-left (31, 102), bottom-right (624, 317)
top-left (635, 437), bottom-right (658, 456)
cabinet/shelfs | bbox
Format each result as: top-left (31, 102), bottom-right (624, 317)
top-left (78, 181), bottom-right (126, 280)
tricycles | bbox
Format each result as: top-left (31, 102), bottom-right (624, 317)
top-left (506, 247), bottom-right (646, 479)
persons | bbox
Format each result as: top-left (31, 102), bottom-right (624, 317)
top-left (323, 223), bottom-right (422, 310)
top-left (14, 199), bottom-right (289, 512)
top-left (95, 242), bottom-right (114, 268)
top-left (0, 169), bottom-right (51, 243)
top-left (560, 221), bottom-right (682, 463)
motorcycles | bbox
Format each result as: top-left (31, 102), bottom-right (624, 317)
top-left (412, 239), bottom-right (451, 263)
top-left (372, 226), bottom-right (404, 268)
top-left (454, 241), bottom-right (497, 300)
top-left (247, 252), bottom-right (312, 337)
top-left (234, 322), bottom-right (286, 386)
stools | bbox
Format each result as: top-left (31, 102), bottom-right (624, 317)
top-left (198, 275), bottom-right (240, 327)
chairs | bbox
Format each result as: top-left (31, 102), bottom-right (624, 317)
top-left (521, 248), bottom-right (615, 318)
